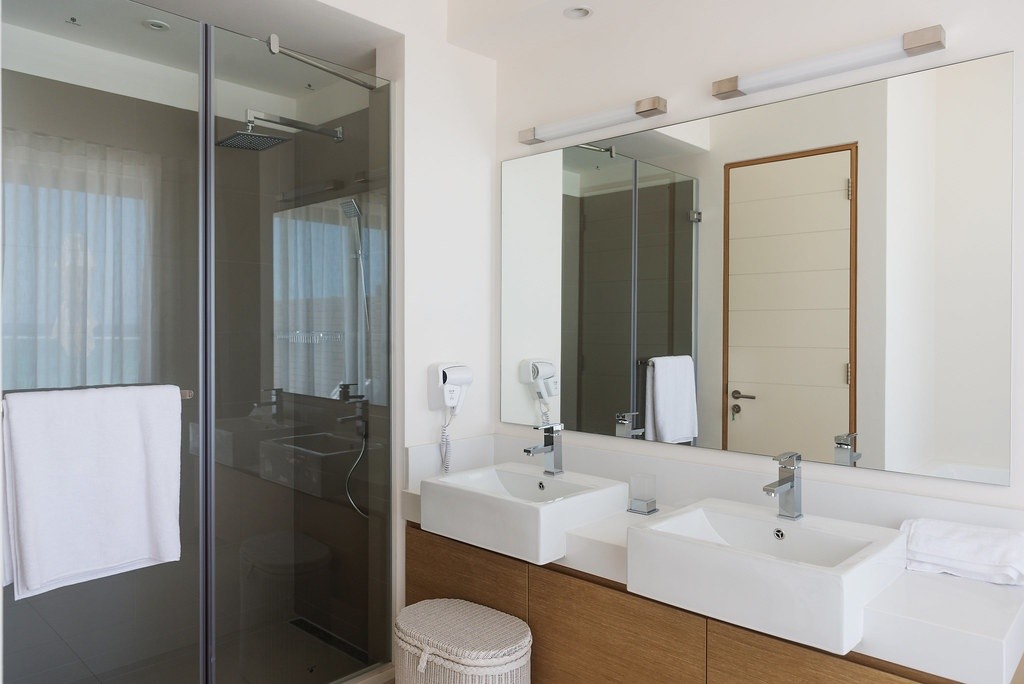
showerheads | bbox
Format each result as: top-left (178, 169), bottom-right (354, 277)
top-left (339, 197), bottom-right (369, 262)
top-left (213, 130), bottom-right (293, 154)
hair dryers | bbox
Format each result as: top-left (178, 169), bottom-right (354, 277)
top-left (443, 365), bottom-right (474, 417)
top-left (530, 363), bottom-right (556, 410)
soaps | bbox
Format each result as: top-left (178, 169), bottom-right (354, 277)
top-left (632, 497), bottom-right (657, 512)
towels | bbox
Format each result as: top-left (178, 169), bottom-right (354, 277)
top-left (1, 382), bottom-right (183, 602)
top-left (644, 355), bottom-right (699, 448)
top-left (898, 517), bottom-right (1024, 588)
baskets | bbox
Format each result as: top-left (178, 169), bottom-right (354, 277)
top-left (394, 598), bottom-right (532, 684)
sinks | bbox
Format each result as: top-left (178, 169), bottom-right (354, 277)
top-left (624, 496), bottom-right (909, 656)
top-left (416, 461), bottom-right (631, 568)
top-left (257, 430), bottom-right (386, 510)
top-left (186, 413), bottom-right (308, 471)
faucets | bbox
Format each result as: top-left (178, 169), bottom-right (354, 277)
top-left (762, 451), bottom-right (805, 519)
top-left (522, 422), bottom-right (567, 475)
top-left (253, 386), bottom-right (286, 422)
top-left (335, 381), bottom-right (367, 404)
top-left (614, 411), bottom-right (646, 439)
top-left (832, 430), bottom-right (862, 467)
top-left (336, 397), bottom-right (372, 437)
